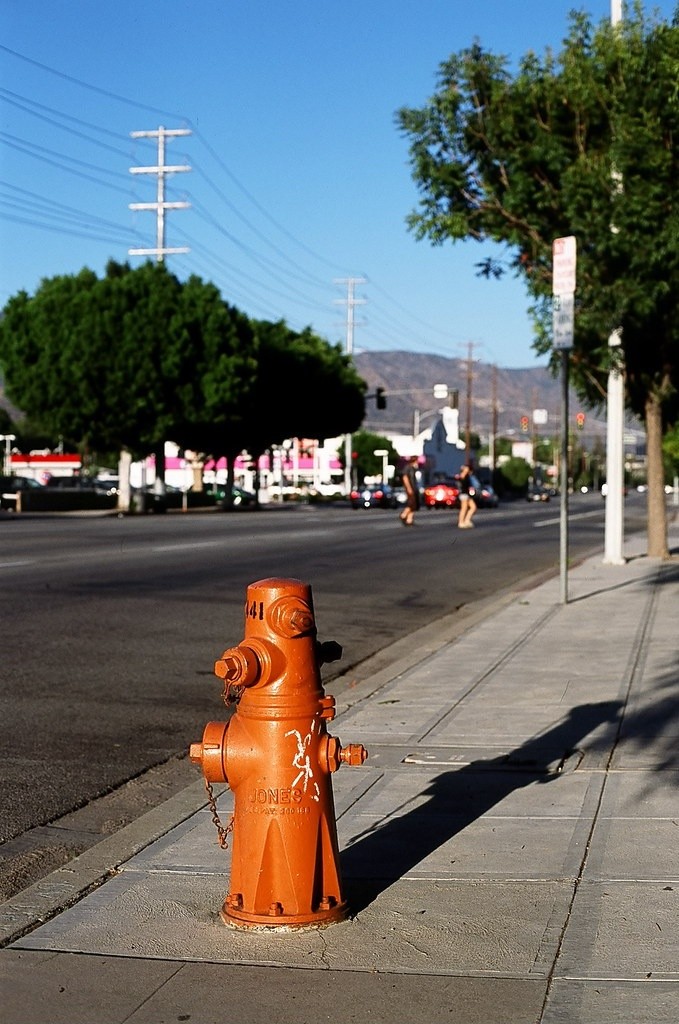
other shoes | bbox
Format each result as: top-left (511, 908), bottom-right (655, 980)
top-left (458, 519), bottom-right (474, 528)
top-left (399, 512), bottom-right (414, 527)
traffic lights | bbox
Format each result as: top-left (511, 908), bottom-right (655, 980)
top-left (520, 416), bottom-right (529, 434)
top-left (577, 412), bottom-right (585, 430)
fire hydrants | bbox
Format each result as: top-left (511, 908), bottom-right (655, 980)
top-left (191, 576), bottom-right (367, 934)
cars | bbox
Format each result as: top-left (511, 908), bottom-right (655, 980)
top-left (526, 487), bottom-right (549, 502)
top-left (0, 476), bottom-right (499, 510)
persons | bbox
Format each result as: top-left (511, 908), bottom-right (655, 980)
top-left (399, 456), bottom-right (422, 529)
top-left (458, 465), bottom-right (478, 529)
